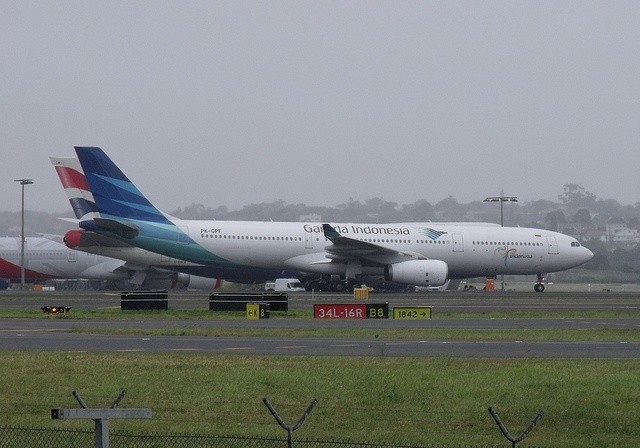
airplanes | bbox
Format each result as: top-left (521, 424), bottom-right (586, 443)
top-left (50, 146), bottom-right (596, 293)
top-left (0, 233), bottom-right (221, 290)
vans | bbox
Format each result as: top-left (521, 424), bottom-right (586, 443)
top-left (276, 277), bottom-right (306, 292)
top-left (265, 282), bottom-right (275, 291)
